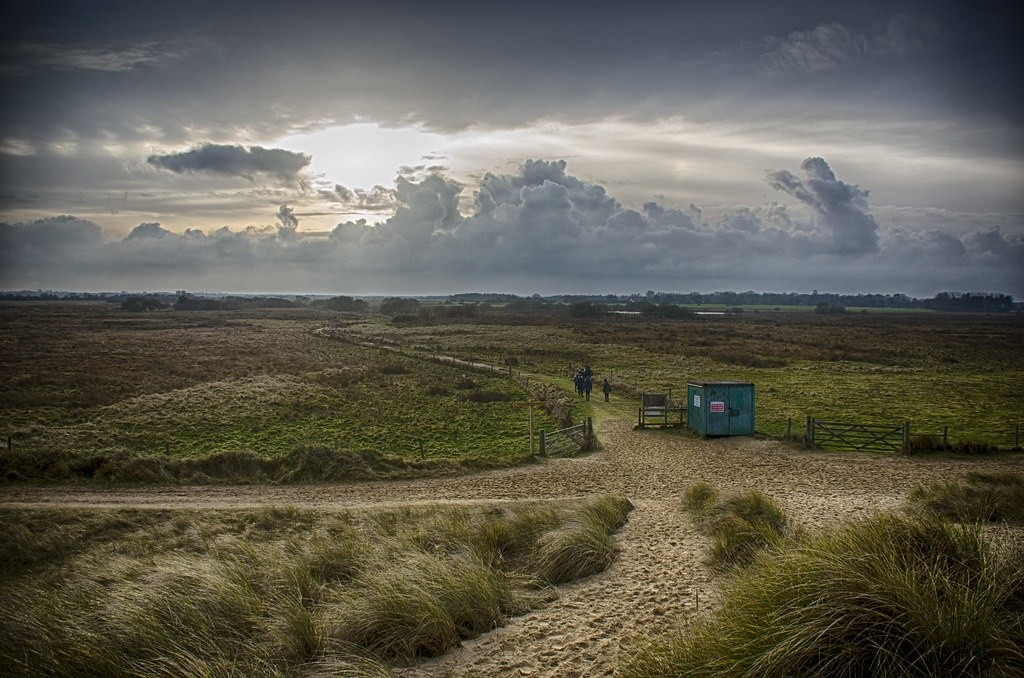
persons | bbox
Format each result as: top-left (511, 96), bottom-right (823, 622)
top-left (574, 366), bottom-right (594, 402)
top-left (602, 378), bottom-right (612, 402)
